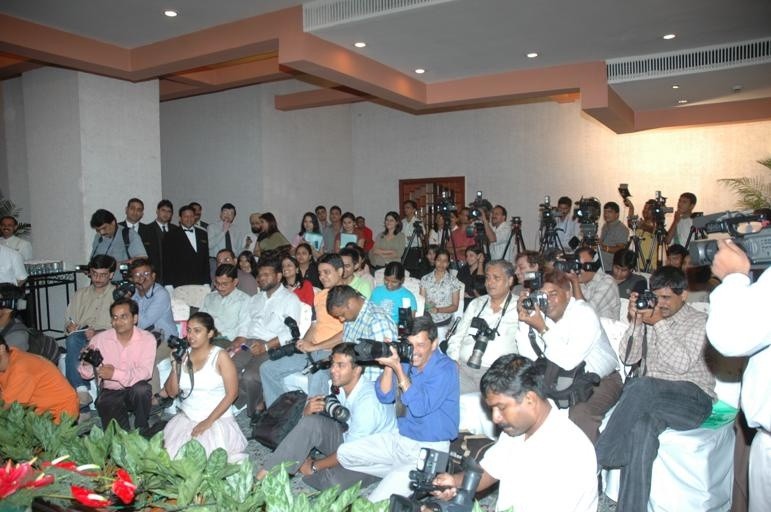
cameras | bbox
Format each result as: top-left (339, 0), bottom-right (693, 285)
top-left (467, 317), bottom-right (495, 369)
top-left (553, 254), bottom-right (598, 275)
top-left (512, 216), bottom-right (522, 226)
top-left (354, 308), bottom-right (414, 366)
top-left (111, 263), bottom-right (136, 300)
top-left (652, 191), bottom-right (673, 223)
top-left (690, 212), bottom-right (703, 219)
top-left (268, 316), bottom-right (304, 361)
top-left (312, 385), bottom-right (350, 423)
top-left (521, 272), bottom-right (549, 316)
top-left (636, 290), bottom-right (658, 309)
top-left (77, 347), bottom-right (103, 367)
top-left (167, 335), bottom-right (190, 362)
top-left (76, 264), bottom-right (91, 278)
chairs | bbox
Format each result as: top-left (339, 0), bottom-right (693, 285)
top-left (284, 272), bottom-right (740, 512)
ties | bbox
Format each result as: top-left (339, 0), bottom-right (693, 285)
top-left (132, 224), bottom-right (135, 230)
top-left (225, 230), bottom-right (232, 249)
top-left (162, 225), bottom-right (166, 231)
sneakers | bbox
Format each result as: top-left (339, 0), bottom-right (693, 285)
top-left (78, 391), bottom-right (93, 409)
top-left (231, 404), bottom-right (247, 417)
top-left (149, 392), bottom-right (174, 415)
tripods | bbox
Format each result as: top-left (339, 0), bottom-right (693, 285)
top-left (539, 228), bottom-right (563, 255)
top-left (624, 234), bottom-right (645, 274)
top-left (645, 222), bottom-right (668, 273)
top-left (502, 228), bottom-right (526, 261)
top-left (684, 225), bottom-right (706, 250)
top-left (401, 221), bottom-right (428, 274)
top-left (440, 219), bottom-right (459, 271)
top-left (474, 231), bottom-right (491, 260)
top-left (579, 223), bottom-right (605, 273)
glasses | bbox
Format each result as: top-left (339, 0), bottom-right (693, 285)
top-left (214, 279), bottom-right (234, 286)
top-left (130, 271), bottom-right (152, 279)
top-left (91, 269), bottom-right (111, 280)
top-left (216, 257), bottom-right (233, 263)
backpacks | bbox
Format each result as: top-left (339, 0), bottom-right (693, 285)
top-left (5, 322), bottom-right (60, 365)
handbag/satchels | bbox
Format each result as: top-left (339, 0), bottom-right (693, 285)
top-left (252, 390), bottom-right (308, 449)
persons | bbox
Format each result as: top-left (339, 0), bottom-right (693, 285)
top-left (0, 193), bottom-right (770, 512)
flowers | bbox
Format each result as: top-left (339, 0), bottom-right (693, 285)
top-left (0, 400), bottom-right (391, 512)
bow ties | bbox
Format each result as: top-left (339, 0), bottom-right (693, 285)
top-left (183, 229), bottom-right (193, 232)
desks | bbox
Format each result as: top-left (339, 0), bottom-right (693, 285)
top-left (15, 270), bottom-right (81, 353)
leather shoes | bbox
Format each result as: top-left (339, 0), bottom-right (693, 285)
top-left (249, 401), bottom-right (267, 425)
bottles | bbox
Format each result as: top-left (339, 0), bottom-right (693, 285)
top-left (23, 262), bottom-right (64, 283)
